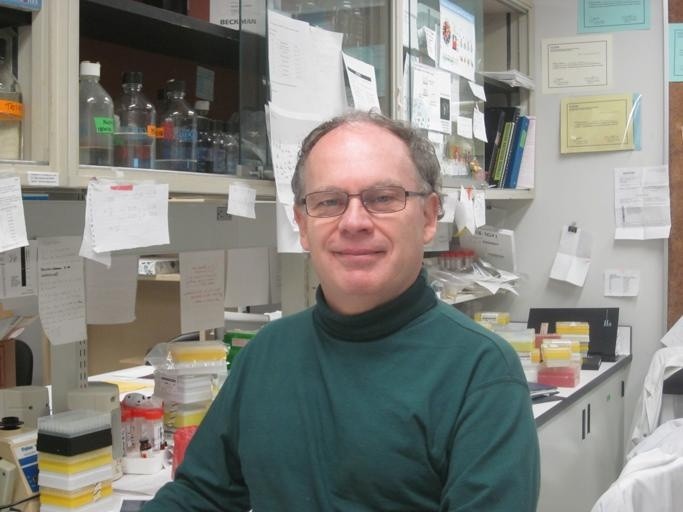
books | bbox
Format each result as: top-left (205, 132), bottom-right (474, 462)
top-left (484, 68), bottom-right (535, 92)
top-left (483, 105), bottom-right (535, 191)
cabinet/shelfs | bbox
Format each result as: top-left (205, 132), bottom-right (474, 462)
top-left (508, 365), bottom-right (632, 512)
top-left (0, 0), bottom-right (535, 201)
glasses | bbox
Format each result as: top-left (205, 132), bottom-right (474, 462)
top-left (298, 185), bottom-right (431, 220)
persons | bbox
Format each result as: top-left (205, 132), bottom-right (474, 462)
top-left (138, 103), bottom-right (543, 512)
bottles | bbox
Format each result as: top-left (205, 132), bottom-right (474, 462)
top-left (334, 0), bottom-right (365, 61)
top-left (79, 59), bottom-right (239, 175)
top-left (438, 251), bottom-right (475, 269)
top-left (0, 38), bottom-right (22, 161)
